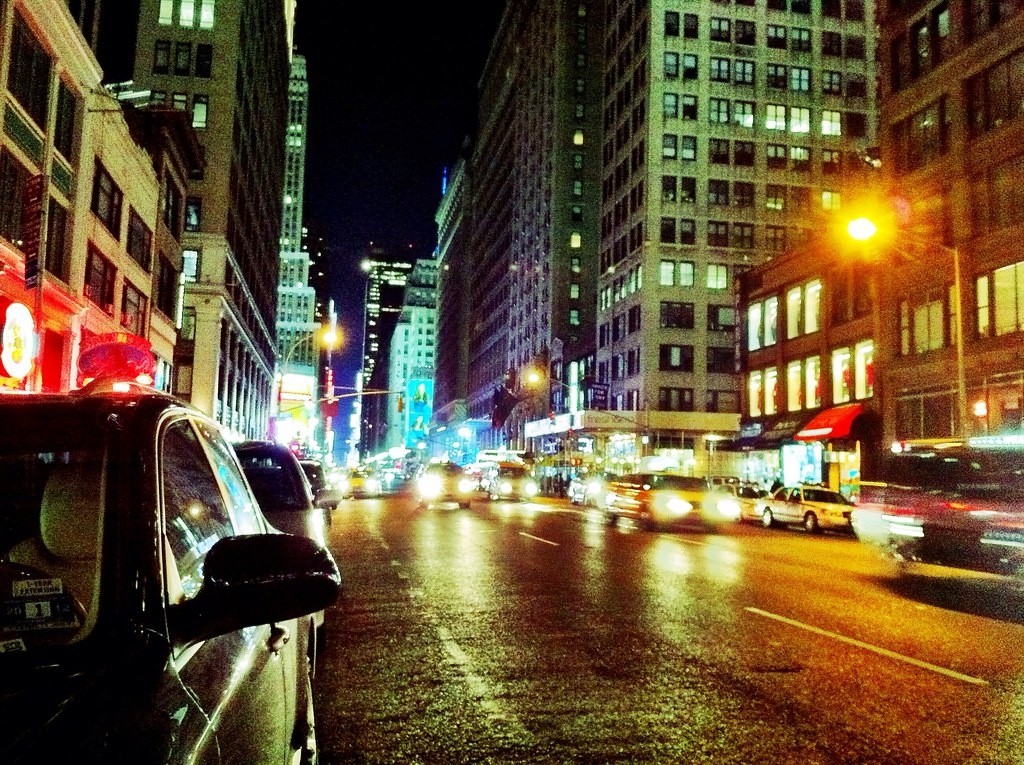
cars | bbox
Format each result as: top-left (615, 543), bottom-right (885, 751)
top-left (604, 473), bottom-right (733, 533)
top-left (566, 479), bottom-right (605, 508)
top-left (0, 374), bottom-right (346, 765)
top-left (419, 462), bottom-right (470, 510)
top-left (851, 440), bottom-right (1023, 580)
top-left (755, 486), bottom-right (856, 530)
top-left (246, 435), bottom-right (410, 674)
top-left (710, 484), bottom-right (764, 522)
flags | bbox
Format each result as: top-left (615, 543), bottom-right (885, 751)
top-left (490, 386), bottom-right (518, 430)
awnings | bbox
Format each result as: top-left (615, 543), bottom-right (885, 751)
top-left (752, 409), bottom-right (820, 446)
top-left (795, 402), bottom-right (863, 441)
top-left (711, 419), bottom-right (772, 451)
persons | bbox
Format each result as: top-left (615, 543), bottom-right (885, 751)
top-left (770, 477), bottom-right (785, 499)
top-left (546, 473), bottom-right (572, 497)
top-left (413, 382), bottom-right (427, 403)
top-left (413, 416), bottom-right (424, 430)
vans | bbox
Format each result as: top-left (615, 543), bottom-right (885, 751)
top-left (483, 462), bottom-right (531, 501)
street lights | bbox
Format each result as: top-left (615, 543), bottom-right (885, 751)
top-left (275, 329), bottom-right (338, 433)
top-left (847, 215), bottom-right (969, 438)
